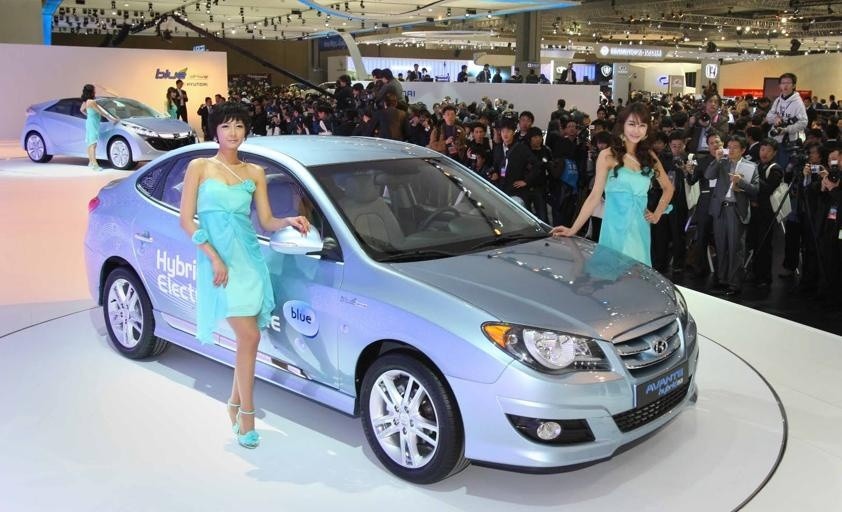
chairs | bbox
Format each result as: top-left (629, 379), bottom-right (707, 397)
top-left (252, 171), bottom-right (310, 245)
top-left (339, 170), bottom-right (410, 254)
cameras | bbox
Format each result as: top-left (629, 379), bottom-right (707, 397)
top-left (810, 165), bottom-right (820, 173)
top-left (828, 159), bottom-right (840, 182)
top-left (770, 115), bottom-right (797, 137)
top-left (790, 150), bottom-right (808, 174)
top-left (694, 112), bottom-right (710, 128)
top-left (451, 129), bottom-right (461, 143)
top-left (485, 166), bottom-right (496, 178)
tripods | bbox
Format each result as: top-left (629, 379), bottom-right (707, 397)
top-left (743, 173), bottom-right (831, 289)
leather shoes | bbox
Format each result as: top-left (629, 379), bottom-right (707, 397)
top-left (723, 288), bottom-right (741, 295)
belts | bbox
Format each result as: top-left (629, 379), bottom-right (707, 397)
top-left (722, 201), bottom-right (736, 207)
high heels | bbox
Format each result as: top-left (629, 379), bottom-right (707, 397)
top-left (227, 399), bottom-right (239, 431)
top-left (235, 407), bottom-right (259, 449)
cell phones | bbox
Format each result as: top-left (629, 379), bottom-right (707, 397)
top-left (720, 148), bottom-right (729, 156)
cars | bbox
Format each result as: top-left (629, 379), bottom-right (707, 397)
top-left (20, 97), bottom-right (197, 167)
top-left (83, 136), bottom-right (697, 482)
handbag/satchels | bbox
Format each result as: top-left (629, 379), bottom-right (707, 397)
top-left (765, 163), bottom-right (792, 223)
top-left (684, 153), bottom-right (701, 210)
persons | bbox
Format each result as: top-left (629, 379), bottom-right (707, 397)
top-left (165, 80), bottom-right (188, 125)
top-left (194, 62), bottom-right (842, 326)
top-left (179, 100), bottom-right (311, 452)
top-left (78, 84), bottom-right (120, 169)
